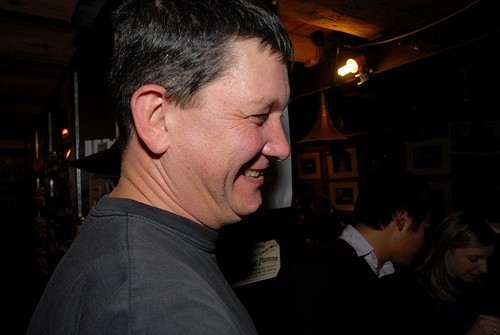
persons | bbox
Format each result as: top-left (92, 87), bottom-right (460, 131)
top-left (254, 166), bottom-right (436, 335)
top-left (24, 0), bottom-right (297, 335)
top-left (392, 205), bottom-right (500, 335)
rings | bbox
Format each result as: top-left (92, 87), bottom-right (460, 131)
top-left (492, 326), bottom-right (497, 334)
